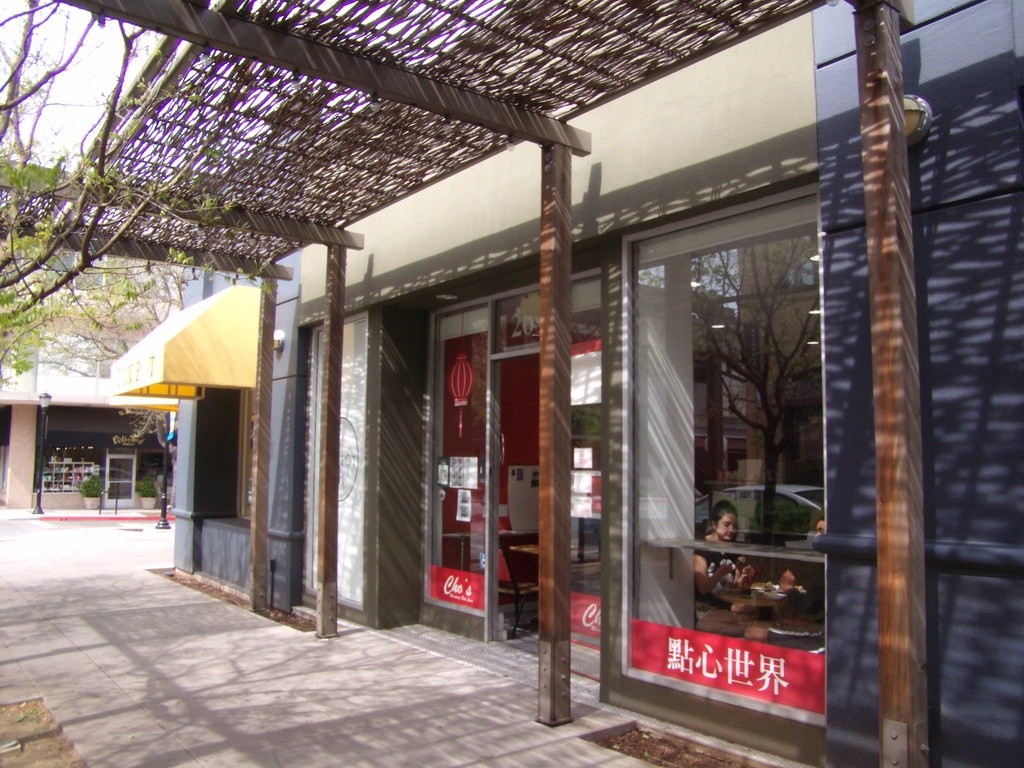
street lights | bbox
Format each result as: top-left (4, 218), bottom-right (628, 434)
top-left (28, 391), bottom-right (52, 516)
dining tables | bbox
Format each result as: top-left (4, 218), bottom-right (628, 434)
top-left (717, 581), bottom-right (792, 615)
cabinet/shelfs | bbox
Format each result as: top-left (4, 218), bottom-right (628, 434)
top-left (41, 462), bottom-right (94, 492)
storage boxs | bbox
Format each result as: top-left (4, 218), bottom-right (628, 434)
top-left (63, 458), bottom-right (72, 462)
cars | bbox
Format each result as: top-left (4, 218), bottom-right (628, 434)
top-left (694, 483), bottom-right (825, 547)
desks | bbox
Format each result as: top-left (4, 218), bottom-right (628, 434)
top-left (509, 538), bottom-right (599, 556)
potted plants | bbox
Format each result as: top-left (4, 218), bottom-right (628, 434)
top-left (139, 475), bottom-right (158, 509)
top-left (82, 475), bottom-right (103, 509)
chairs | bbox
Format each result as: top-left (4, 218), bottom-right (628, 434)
top-left (497, 544), bottom-right (539, 641)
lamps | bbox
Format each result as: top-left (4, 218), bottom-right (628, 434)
top-left (903, 95), bottom-right (931, 146)
top-left (273, 328), bottom-right (286, 351)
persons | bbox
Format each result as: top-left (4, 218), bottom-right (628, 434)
top-left (766, 516), bottom-right (826, 654)
top-left (693, 499), bottom-right (770, 642)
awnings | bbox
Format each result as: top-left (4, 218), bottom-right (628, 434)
top-left (111, 286), bottom-right (262, 401)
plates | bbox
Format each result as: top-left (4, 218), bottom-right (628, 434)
top-left (751, 587), bottom-right (774, 590)
